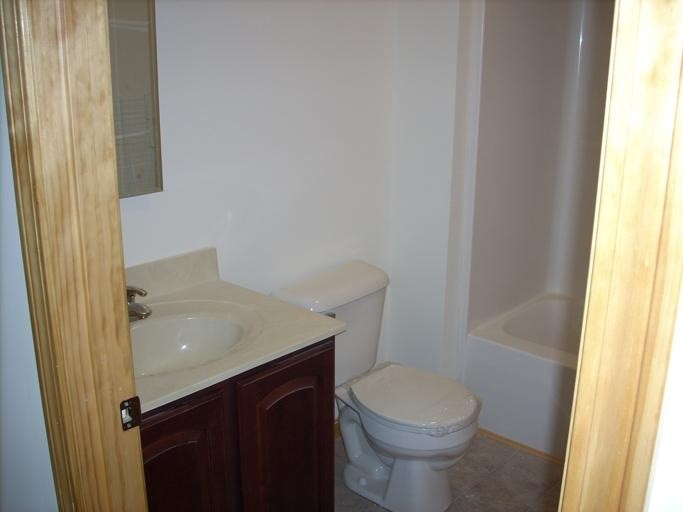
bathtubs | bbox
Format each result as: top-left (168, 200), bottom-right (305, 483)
top-left (466, 292), bottom-right (590, 461)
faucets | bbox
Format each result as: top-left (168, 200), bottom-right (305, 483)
top-left (127, 287), bottom-right (151, 321)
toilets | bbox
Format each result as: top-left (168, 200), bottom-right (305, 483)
top-left (272, 260), bottom-right (479, 512)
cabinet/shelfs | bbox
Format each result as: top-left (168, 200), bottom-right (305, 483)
top-left (135, 336), bottom-right (336, 512)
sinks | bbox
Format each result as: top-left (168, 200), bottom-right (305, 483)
top-left (130, 314), bottom-right (247, 379)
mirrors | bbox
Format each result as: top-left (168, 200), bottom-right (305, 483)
top-left (108, 1), bottom-right (163, 200)
